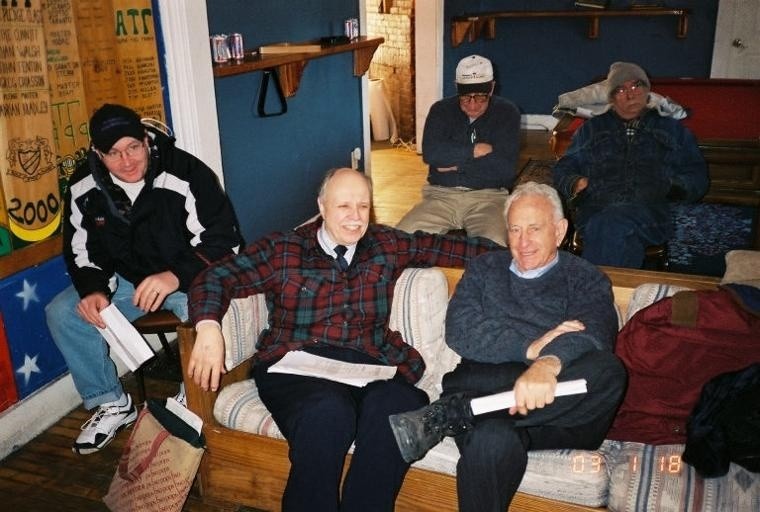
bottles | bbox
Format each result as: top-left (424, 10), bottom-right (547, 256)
top-left (456, 55), bottom-right (494, 96)
top-left (90, 103), bottom-right (145, 154)
top-left (606, 62), bottom-right (650, 103)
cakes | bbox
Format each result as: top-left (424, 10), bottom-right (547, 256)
top-left (345, 19), bottom-right (359, 41)
top-left (212, 34), bottom-right (243, 61)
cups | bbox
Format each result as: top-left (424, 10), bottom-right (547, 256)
top-left (457, 94), bottom-right (489, 105)
top-left (99, 139), bottom-right (146, 162)
top-left (615, 81), bottom-right (645, 96)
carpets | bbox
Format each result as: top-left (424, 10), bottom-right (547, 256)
top-left (334, 245), bottom-right (348, 272)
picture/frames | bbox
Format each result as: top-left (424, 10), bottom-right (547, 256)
top-left (546, 78), bottom-right (760, 207)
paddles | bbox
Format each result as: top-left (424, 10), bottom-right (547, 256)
top-left (510, 157), bottom-right (759, 279)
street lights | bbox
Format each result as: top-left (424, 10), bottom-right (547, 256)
top-left (102, 401), bottom-right (207, 512)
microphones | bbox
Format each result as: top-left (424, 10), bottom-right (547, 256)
top-left (568, 223), bottom-right (669, 270)
top-left (131, 309), bottom-right (184, 401)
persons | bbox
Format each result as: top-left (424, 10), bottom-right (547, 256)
top-left (188, 167), bottom-right (512, 511)
top-left (395, 53), bottom-right (521, 249)
top-left (552, 61), bottom-right (711, 269)
top-left (44, 100), bottom-right (247, 455)
top-left (388, 181), bottom-right (629, 511)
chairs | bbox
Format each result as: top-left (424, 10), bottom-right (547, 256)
top-left (71, 392), bottom-right (138, 456)
top-left (174, 382), bottom-right (188, 408)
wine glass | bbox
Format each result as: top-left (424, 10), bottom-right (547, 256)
top-left (148, 289), bottom-right (157, 296)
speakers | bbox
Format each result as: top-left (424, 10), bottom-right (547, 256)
top-left (389, 393), bottom-right (477, 463)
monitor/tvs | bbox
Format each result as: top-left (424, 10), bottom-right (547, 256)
top-left (259, 40), bottom-right (323, 55)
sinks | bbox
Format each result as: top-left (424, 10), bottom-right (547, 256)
top-left (177, 264), bottom-right (759, 512)
